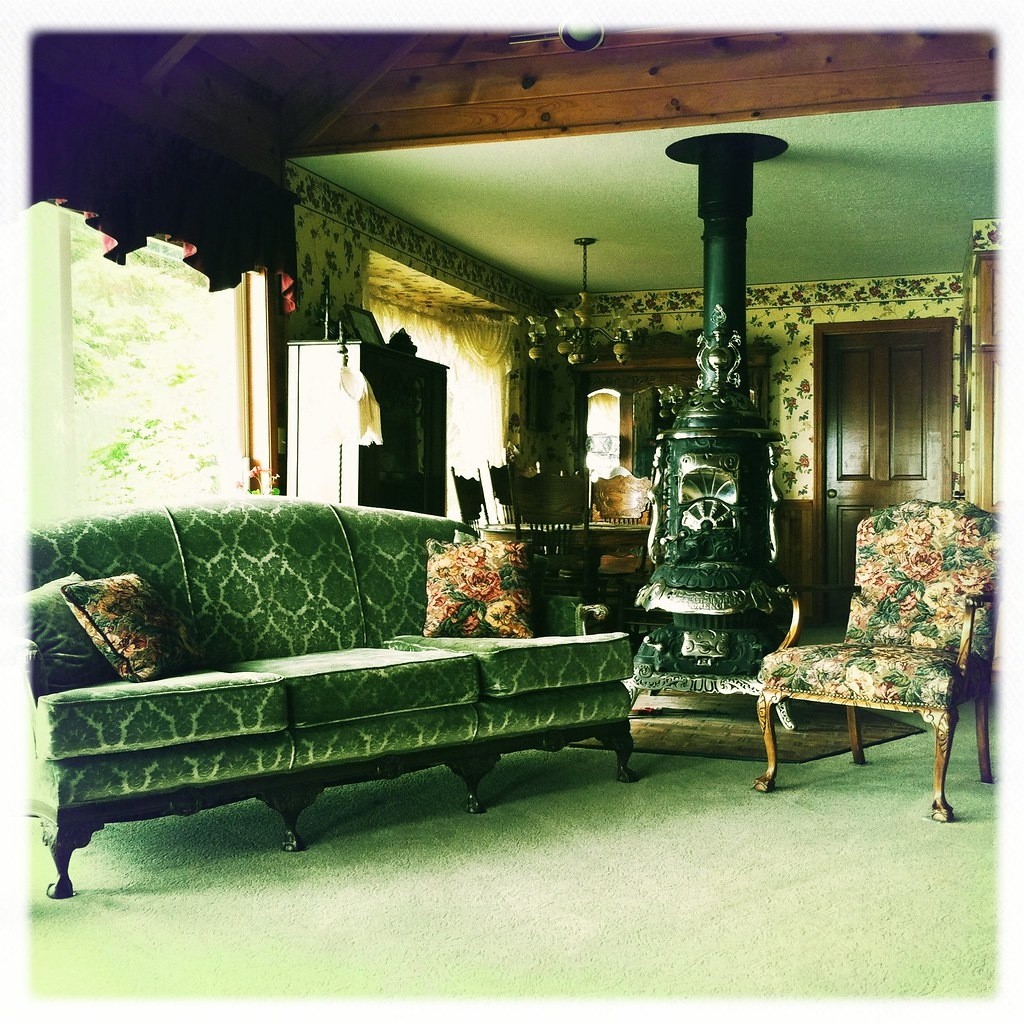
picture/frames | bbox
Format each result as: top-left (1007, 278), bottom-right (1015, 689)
top-left (343, 303), bottom-right (386, 347)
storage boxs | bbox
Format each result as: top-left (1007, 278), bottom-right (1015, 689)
top-left (962, 218), bottom-right (1002, 288)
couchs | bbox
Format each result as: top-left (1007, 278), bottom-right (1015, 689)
top-left (21, 494), bottom-right (640, 900)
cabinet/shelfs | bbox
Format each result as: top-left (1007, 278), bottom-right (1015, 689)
top-left (959, 251), bottom-right (1001, 513)
top-left (286, 340), bottom-right (450, 518)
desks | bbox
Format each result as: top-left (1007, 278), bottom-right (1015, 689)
top-left (481, 522), bottom-right (649, 605)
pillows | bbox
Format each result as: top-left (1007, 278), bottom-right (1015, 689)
top-left (423, 538), bottom-right (534, 640)
top-left (60, 570), bottom-right (203, 682)
top-left (455, 530), bottom-right (548, 592)
top-left (21, 572), bottom-right (117, 694)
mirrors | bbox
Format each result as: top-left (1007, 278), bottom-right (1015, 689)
top-left (632, 384), bottom-right (756, 480)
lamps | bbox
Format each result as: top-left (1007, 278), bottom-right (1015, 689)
top-left (657, 386), bottom-right (688, 418)
top-left (525, 238), bottom-right (633, 366)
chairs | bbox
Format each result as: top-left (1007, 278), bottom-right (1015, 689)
top-left (757, 500), bottom-right (1001, 823)
top-left (451, 459), bottom-right (655, 651)
